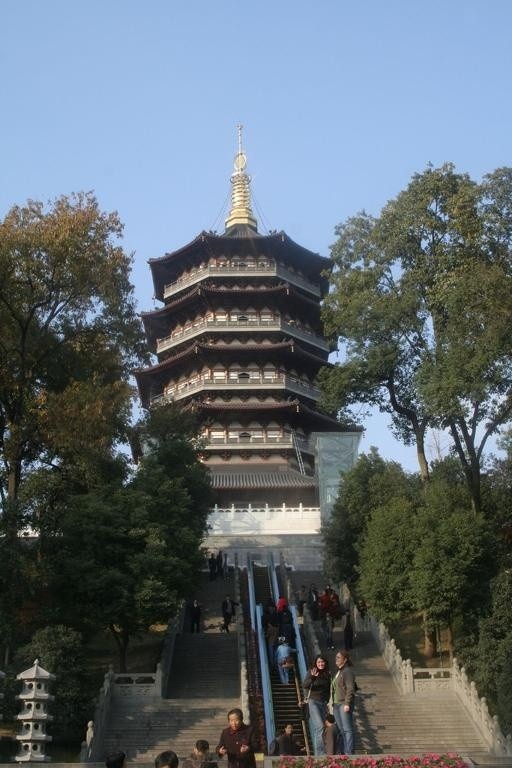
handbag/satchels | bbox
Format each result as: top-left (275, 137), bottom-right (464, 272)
top-left (303, 702), bottom-right (310, 721)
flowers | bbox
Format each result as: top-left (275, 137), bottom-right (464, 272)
top-left (276, 750), bottom-right (468, 768)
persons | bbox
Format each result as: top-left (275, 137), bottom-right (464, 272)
top-left (154, 750), bottom-right (179, 768)
top-left (219, 594), bottom-right (239, 633)
top-left (261, 584), bottom-right (353, 685)
top-left (208, 549), bottom-right (227, 580)
top-left (183, 740), bottom-right (218, 767)
top-left (106, 750), bottom-right (126, 767)
top-left (190, 598), bottom-right (201, 633)
top-left (216, 708), bottom-right (260, 767)
top-left (303, 650), bottom-right (356, 756)
top-left (269, 723), bottom-right (297, 756)
